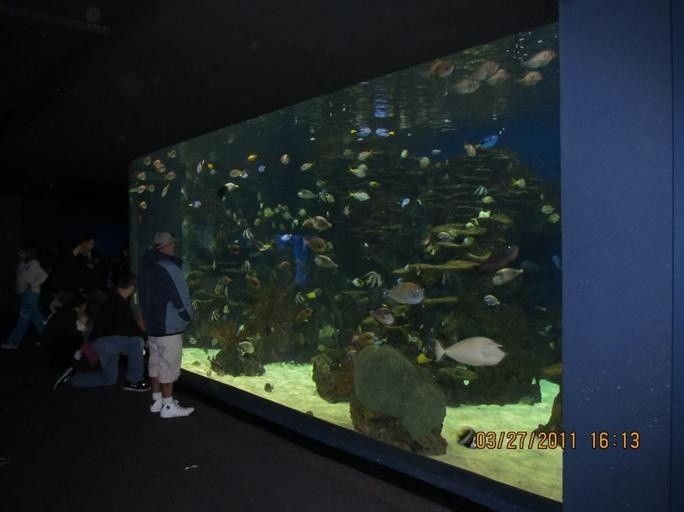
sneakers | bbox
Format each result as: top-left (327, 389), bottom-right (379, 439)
top-left (2, 344), bottom-right (18, 349)
top-left (150, 399), bottom-right (179, 412)
top-left (123, 381), bottom-right (151, 391)
top-left (160, 403), bottom-right (195, 418)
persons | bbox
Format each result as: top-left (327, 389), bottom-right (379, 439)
top-left (138, 232), bottom-right (195, 419)
top-left (1, 231), bottom-right (150, 392)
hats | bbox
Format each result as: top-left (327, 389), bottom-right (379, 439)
top-left (154, 233), bottom-right (179, 248)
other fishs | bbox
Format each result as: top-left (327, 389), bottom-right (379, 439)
top-left (450, 425), bottom-right (481, 449)
top-left (129, 48), bottom-right (561, 394)
